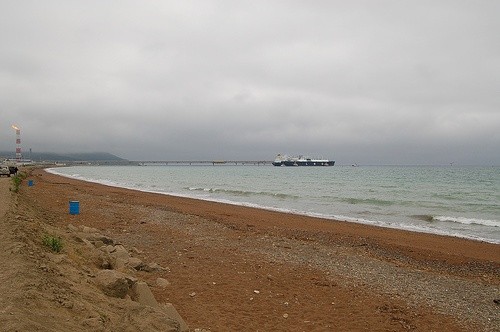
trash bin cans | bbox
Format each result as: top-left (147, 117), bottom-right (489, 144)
top-left (29, 180), bottom-right (33, 186)
top-left (70, 201), bottom-right (80, 215)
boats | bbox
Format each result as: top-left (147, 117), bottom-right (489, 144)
top-left (271, 157), bottom-right (335, 166)
